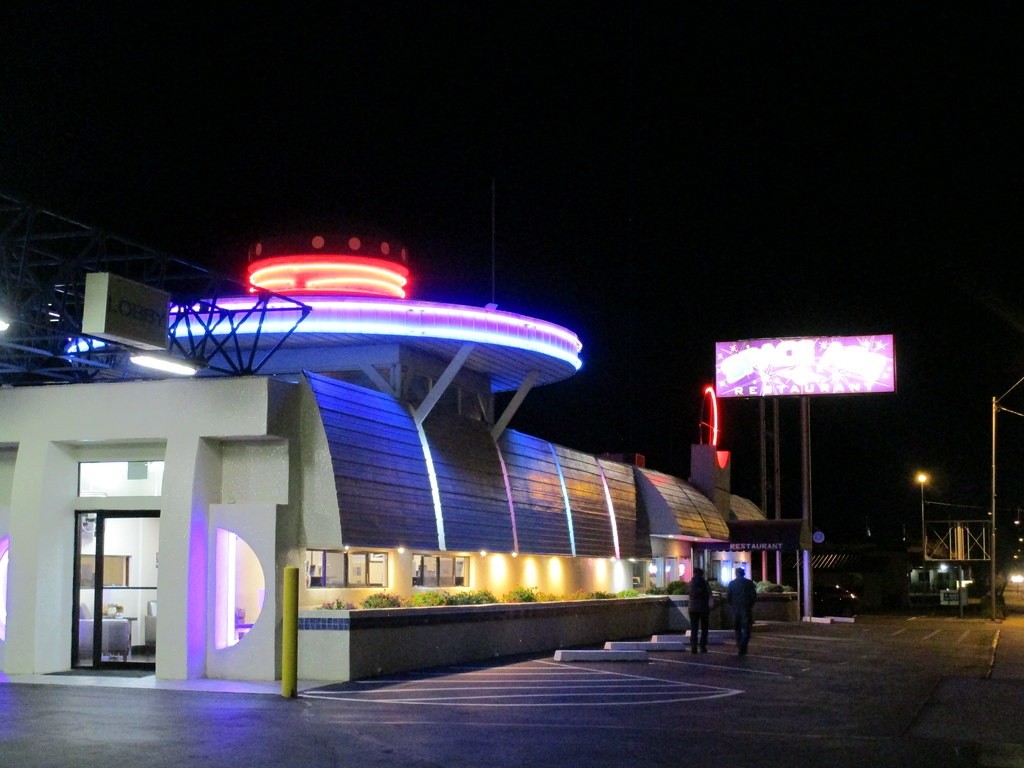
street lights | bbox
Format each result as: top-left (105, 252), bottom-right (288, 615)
top-left (918, 474), bottom-right (927, 613)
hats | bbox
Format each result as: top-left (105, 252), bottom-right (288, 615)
top-left (736, 568), bottom-right (745, 574)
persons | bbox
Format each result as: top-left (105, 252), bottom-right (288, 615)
top-left (727, 567), bottom-right (755, 654)
top-left (686, 567), bottom-right (714, 655)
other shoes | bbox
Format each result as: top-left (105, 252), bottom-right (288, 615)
top-left (735, 642), bottom-right (746, 654)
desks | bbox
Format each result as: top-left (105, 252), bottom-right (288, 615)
top-left (79, 616), bottom-right (131, 661)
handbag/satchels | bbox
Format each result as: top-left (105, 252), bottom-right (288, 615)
top-left (707, 584), bottom-right (720, 610)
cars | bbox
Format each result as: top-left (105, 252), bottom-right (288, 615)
top-left (800, 584), bottom-right (861, 616)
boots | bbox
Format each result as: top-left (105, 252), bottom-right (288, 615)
top-left (690, 637), bottom-right (698, 654)
top-left (700, 638), bottom-right (707, 652)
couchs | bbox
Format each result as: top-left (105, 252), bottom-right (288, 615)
top-left (145, 600), bottom-right (156, 656)
